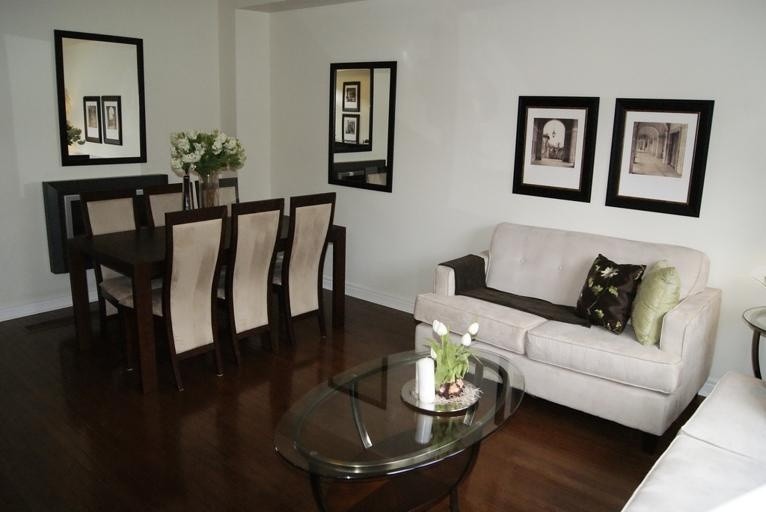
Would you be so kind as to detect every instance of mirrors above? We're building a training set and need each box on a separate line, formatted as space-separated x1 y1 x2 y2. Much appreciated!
327 59 399 195
332 67 373 154
53 27 147 165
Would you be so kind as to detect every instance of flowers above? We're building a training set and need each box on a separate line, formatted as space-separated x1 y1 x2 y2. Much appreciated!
428 319 483 385
170 128 241 176
65 119 85 148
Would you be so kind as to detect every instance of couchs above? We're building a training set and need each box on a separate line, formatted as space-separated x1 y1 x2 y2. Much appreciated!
611 372 766 512
406 221 725 437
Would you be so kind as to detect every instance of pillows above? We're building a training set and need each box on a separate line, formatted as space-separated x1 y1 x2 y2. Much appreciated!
628 264 685 348
572 246 643 337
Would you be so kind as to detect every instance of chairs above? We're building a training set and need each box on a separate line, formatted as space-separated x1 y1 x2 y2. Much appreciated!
31 168 238 338
285 186 337 361
227 195 289 365
96 205 225 394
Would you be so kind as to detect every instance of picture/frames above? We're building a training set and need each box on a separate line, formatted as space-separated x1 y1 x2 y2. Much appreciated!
342 114 360 147
100 96 124 147
603 95 717 219
342 82 361 112
81 92 102 145
510 94 601 203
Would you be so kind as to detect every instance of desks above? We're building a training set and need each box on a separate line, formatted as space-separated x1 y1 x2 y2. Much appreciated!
739 302 766 382
53 218 354 387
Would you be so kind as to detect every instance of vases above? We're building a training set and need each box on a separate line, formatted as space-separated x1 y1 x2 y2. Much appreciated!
192 163 222 210
433 372 468 400
